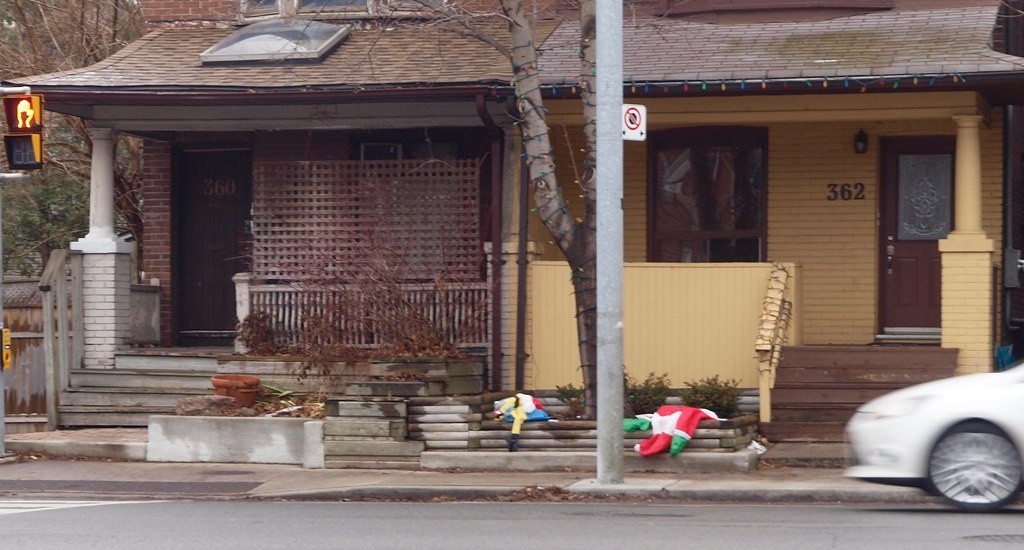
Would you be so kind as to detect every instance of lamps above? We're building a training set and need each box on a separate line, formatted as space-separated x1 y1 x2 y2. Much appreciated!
853 126 870 155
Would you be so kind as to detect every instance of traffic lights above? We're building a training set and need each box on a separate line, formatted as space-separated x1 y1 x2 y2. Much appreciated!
1 93 44 169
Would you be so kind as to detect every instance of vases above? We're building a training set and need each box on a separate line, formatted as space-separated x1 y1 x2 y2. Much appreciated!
211 375 261 409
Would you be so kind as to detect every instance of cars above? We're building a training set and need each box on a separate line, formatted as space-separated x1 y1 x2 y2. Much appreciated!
842 362 1024 513
